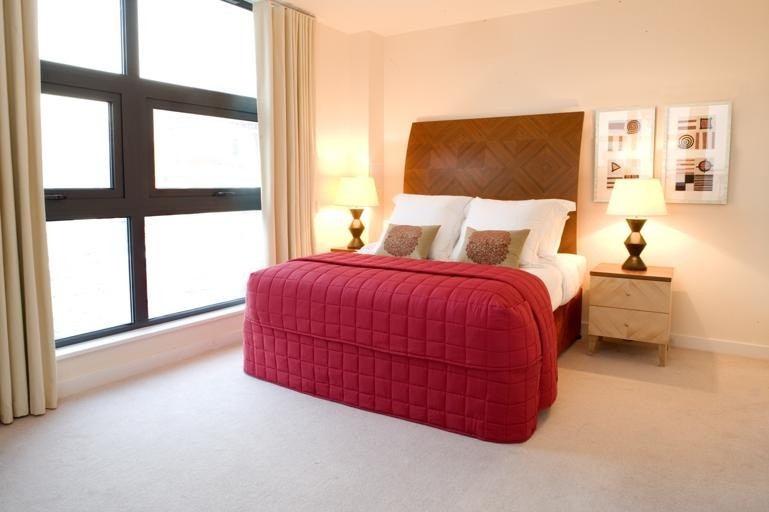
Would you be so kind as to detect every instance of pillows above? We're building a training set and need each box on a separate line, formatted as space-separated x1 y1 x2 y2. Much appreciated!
374 193 570 269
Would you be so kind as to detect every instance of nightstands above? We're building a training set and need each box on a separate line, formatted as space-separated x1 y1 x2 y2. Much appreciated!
585 263 678 367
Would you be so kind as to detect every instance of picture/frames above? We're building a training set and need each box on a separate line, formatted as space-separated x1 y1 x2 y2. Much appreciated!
590 99 732 205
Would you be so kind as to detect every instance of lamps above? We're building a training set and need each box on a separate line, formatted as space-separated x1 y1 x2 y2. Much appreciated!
337 172 380 250
603 179 669 273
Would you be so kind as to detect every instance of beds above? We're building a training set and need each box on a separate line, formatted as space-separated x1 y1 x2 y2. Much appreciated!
247 110 588 442
